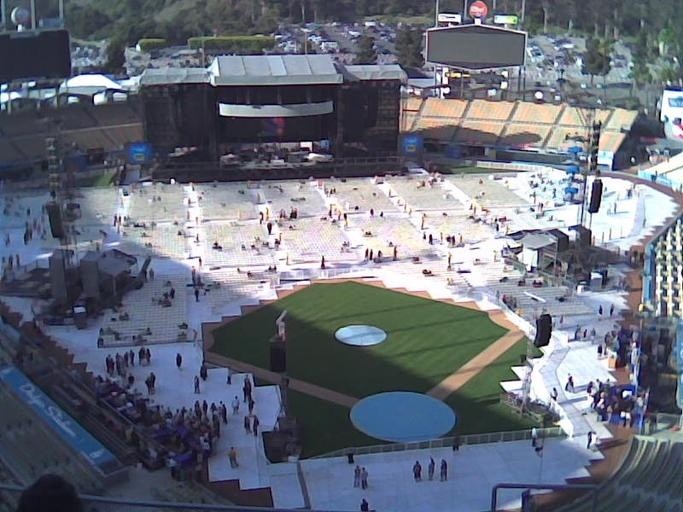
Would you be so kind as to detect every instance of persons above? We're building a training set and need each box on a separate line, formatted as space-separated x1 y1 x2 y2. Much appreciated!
352 464 361 488
259 169 637 294
18 474 82 512
428 455 435 480
413 460 422 482
495 294 649 454
1 183 260 476
360 498 369 511
360 466 368 488
452 434 461 452
439 458 447 481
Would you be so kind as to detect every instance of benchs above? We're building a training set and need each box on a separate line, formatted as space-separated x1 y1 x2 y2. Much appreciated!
0 336 201 479
644 219 683 318
